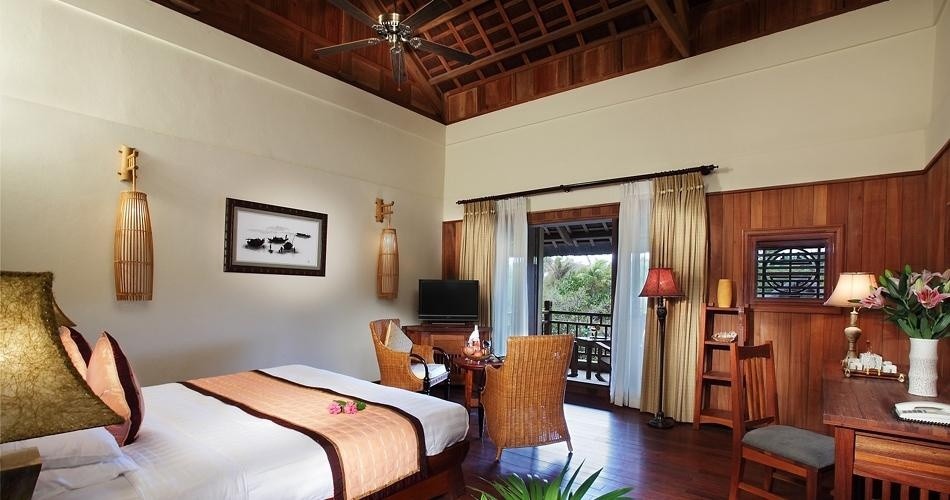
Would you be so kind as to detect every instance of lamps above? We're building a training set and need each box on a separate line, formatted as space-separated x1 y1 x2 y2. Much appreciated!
818 266 887 364
636 264 690 430
372 195 401 300
0 270 129 448
109 141 157 303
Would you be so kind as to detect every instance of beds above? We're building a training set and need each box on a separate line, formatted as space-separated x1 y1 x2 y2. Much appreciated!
0 362 480 497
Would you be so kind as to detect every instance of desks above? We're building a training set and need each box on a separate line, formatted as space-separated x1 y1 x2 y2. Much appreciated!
814 357 948 500
573 335 615 384
451 354 515 438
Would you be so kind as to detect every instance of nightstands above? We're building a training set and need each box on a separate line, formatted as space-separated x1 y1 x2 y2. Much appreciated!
1 443 43 500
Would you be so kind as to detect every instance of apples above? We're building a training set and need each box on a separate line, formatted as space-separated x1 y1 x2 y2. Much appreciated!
463 342 482 357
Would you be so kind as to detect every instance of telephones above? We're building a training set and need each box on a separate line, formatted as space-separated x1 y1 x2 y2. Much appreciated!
895 400 950 427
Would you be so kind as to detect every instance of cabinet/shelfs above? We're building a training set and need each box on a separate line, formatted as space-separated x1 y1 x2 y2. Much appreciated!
689 300 760 435
400 321 493 393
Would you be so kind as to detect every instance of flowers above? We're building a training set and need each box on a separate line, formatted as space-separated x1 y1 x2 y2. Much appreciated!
854 258 949 343
323 394 370 416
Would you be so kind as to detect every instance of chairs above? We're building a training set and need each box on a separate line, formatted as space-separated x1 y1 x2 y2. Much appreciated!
591 339 614 384
719 336 837 499
476 328 577 462
368 317 453 403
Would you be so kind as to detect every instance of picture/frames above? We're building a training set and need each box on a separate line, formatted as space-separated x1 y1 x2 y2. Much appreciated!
223 193 328 279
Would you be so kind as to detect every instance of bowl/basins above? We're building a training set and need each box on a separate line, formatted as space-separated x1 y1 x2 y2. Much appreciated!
463 346 491 362
711 332 737 342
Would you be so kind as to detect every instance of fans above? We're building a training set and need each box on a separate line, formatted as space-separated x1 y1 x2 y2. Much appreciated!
303 1 483 97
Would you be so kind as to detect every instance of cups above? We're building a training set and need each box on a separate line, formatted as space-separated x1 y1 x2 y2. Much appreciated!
717 279 733 308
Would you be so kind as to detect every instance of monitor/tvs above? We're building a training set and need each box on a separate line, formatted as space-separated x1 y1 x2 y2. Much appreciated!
418 279 480 323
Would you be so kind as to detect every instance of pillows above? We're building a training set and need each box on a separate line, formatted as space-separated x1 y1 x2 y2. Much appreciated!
53 315 92 383
1 422 130 476
83 326 145 446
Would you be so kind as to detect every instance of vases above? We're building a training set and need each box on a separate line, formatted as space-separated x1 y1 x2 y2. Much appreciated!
715 276 735 310
904 331 942 400
